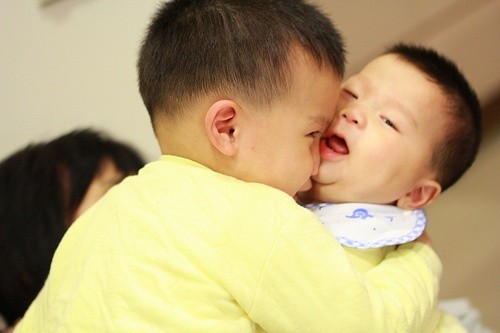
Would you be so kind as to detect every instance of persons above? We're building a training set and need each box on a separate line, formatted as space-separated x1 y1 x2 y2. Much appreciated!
13 0 442 333
0 127 145 333
295 42 484 333
